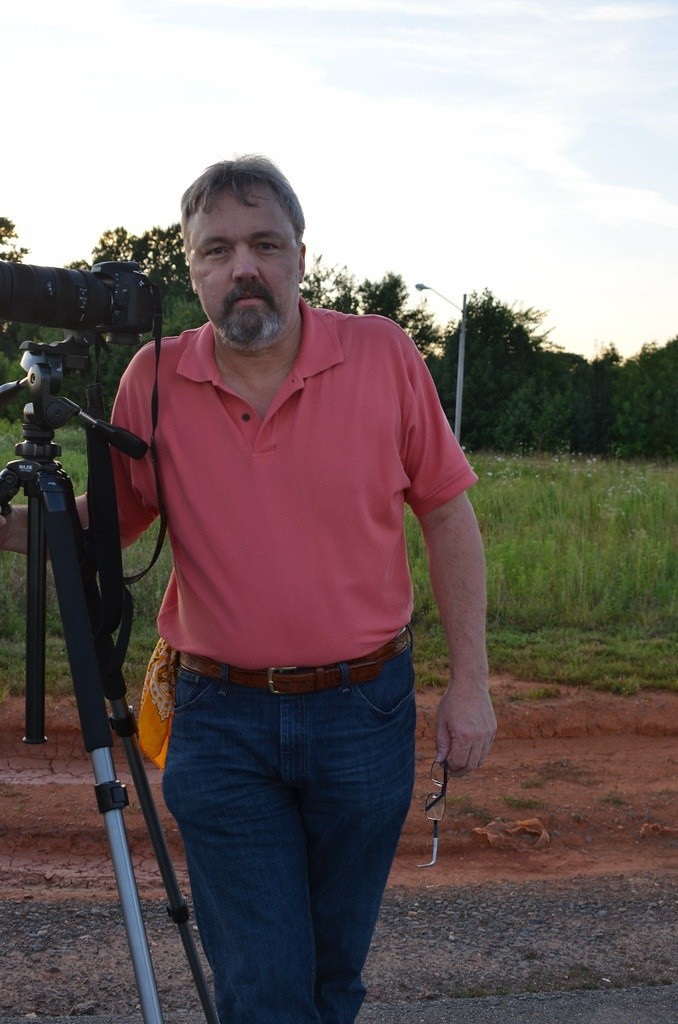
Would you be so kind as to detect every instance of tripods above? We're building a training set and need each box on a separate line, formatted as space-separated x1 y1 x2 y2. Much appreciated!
0 370 221 1024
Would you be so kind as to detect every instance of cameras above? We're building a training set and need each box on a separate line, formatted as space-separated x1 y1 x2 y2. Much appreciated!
0 257 156 345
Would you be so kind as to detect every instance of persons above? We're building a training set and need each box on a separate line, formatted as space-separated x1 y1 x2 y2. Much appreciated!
0 154 498 1024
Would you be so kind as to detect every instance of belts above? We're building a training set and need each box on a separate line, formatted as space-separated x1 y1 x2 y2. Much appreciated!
178 627 410 696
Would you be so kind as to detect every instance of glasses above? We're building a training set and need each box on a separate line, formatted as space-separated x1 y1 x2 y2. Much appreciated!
417 761 451 868
